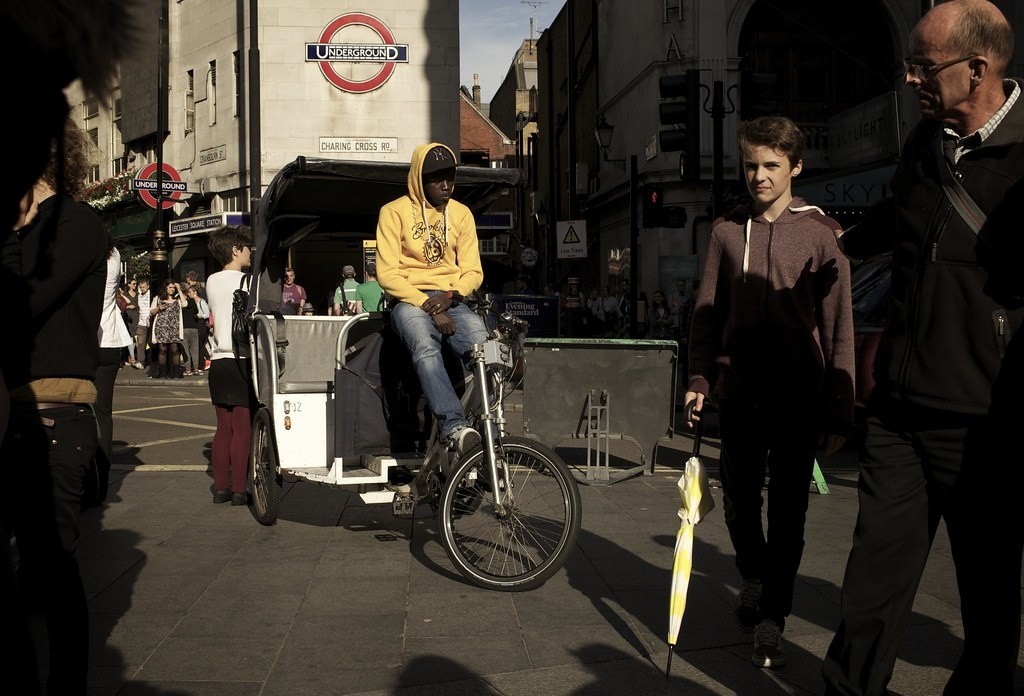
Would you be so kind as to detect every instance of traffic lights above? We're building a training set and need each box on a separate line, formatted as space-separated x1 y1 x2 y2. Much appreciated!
644 185 664 227
656 68 701 182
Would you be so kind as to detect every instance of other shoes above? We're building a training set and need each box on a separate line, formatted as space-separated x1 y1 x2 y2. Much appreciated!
132 361 144 370
183 369 192 376
203 358 211 371
231 493 252 506
193 370 203 376
213 493 232 504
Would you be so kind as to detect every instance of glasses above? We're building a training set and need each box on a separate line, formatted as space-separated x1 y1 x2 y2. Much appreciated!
904 53 986 83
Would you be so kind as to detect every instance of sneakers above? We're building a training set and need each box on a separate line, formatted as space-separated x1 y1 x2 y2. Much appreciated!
735 567 763 632
475 466 513 490
751 620 786 668
451 426 478 458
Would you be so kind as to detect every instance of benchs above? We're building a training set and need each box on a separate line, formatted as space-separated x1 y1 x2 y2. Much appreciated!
258 314 386 394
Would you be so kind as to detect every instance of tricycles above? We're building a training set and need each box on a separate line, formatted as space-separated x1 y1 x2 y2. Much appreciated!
254 155 581 595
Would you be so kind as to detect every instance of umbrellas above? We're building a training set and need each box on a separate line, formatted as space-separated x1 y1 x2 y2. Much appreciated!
666 399 715 677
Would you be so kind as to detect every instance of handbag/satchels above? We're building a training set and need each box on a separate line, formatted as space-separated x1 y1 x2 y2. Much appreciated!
232 278 256 344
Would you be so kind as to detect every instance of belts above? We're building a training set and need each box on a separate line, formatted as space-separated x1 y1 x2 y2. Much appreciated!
12 401 72 412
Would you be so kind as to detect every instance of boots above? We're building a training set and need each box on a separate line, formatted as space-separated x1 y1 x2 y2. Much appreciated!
156 364 167 381
173 364 182 381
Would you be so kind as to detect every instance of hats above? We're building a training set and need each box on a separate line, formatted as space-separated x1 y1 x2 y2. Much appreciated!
422 148 463 175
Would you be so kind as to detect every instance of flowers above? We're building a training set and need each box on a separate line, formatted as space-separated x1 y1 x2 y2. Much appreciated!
127 251 153 280
73 165 139 210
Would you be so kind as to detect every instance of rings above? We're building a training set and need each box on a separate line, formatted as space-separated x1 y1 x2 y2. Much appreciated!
437 304 441 308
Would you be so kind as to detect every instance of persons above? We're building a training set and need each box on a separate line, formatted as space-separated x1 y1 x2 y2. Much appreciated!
822 1 1023 696
205 226 261 506
283 267 307 315
0 0 135 696
375 142 514 493
327 264 385 316
683 115 856 668
516 276 700 390
116 266 209 379
